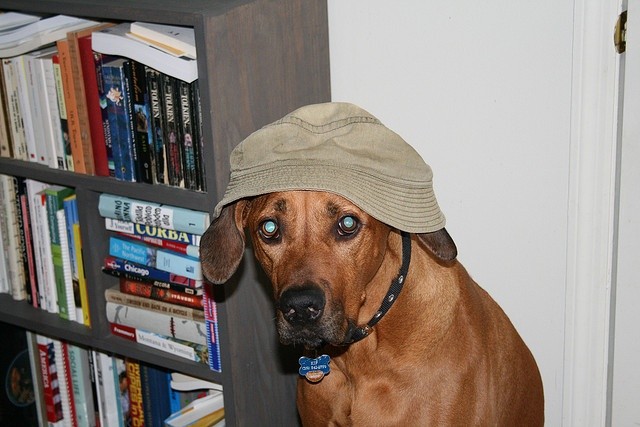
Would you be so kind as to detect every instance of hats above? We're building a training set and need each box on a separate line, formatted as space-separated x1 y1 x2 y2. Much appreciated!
212 102 447 233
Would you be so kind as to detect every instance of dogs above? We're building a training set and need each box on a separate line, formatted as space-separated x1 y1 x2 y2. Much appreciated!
198 102 544 427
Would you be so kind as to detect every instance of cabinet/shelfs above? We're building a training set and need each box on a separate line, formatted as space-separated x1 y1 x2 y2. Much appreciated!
0 2 329 427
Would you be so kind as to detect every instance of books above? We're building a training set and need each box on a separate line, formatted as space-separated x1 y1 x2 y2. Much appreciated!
0 320 227 427
97 192 223 372
0 10 207 194
2 174 89 328
